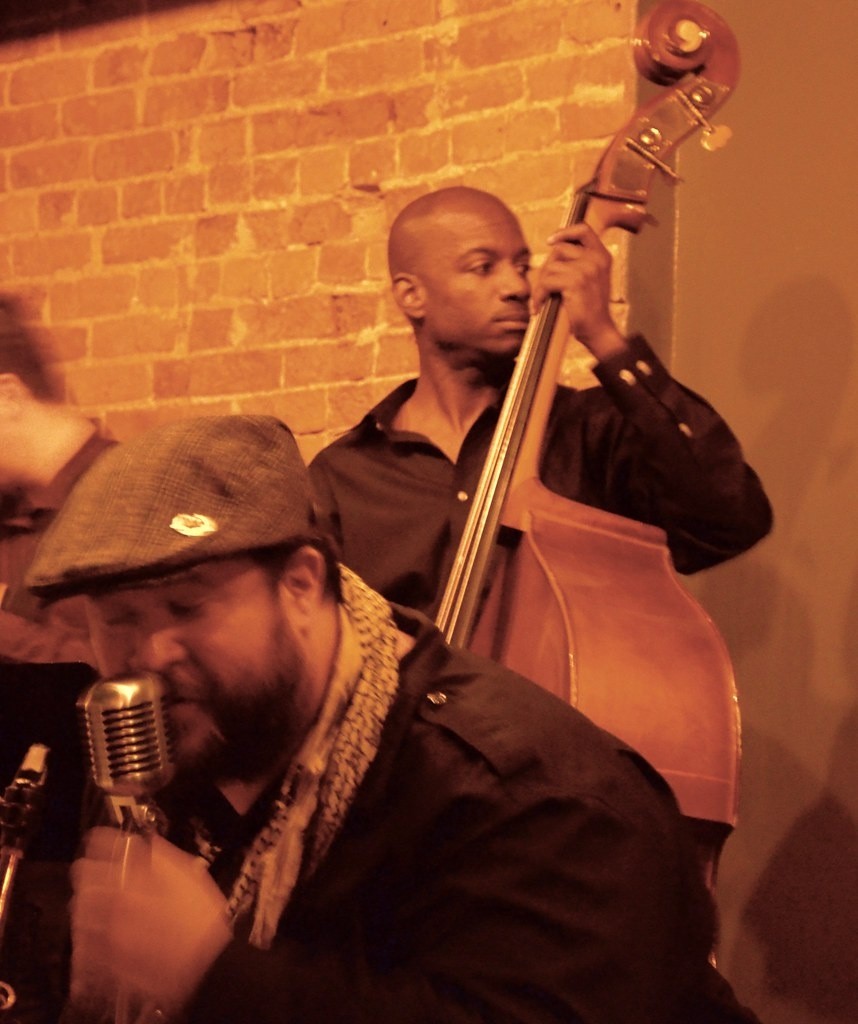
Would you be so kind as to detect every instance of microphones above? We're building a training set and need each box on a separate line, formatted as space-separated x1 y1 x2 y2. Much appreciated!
78 671 175 837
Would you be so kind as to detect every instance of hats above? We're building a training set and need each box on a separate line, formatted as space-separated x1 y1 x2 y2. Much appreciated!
26 415 313 602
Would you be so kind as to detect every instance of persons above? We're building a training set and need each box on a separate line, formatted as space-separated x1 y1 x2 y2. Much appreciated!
0 290 124 676
305 184 776 626
0 412 763 1024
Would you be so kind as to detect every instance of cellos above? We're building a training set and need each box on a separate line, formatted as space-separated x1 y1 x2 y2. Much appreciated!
430 2 743 965
